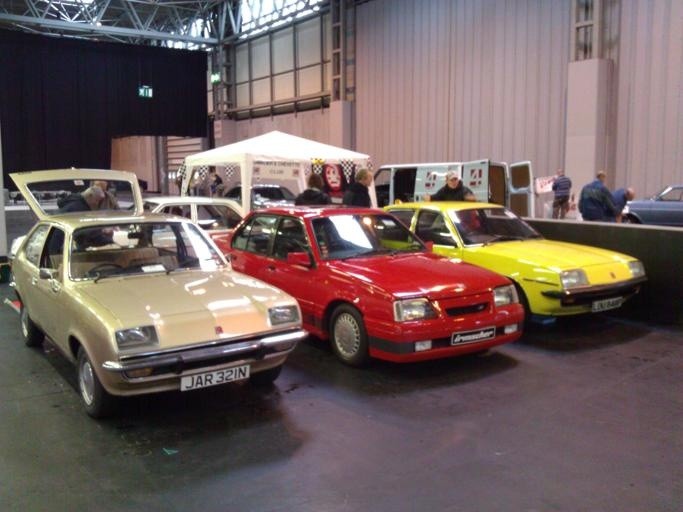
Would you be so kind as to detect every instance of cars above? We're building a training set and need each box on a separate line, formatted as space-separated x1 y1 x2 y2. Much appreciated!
377 200 652 328
620 183 683 228
6 169 310 421
209 203 525 369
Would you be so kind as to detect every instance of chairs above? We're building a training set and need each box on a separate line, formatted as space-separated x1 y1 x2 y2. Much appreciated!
43 248 178 276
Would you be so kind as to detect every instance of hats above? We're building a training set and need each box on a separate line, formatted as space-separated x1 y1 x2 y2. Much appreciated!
446 172 458 181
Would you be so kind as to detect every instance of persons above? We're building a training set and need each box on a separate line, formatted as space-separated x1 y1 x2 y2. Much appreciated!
421 170 477 203
46 185 128 255
291 174 332 228
208 167 224 194
87 180 120 212
610 185 635 224
342 168 374 209
551 168 572 219
578 169 628 222
